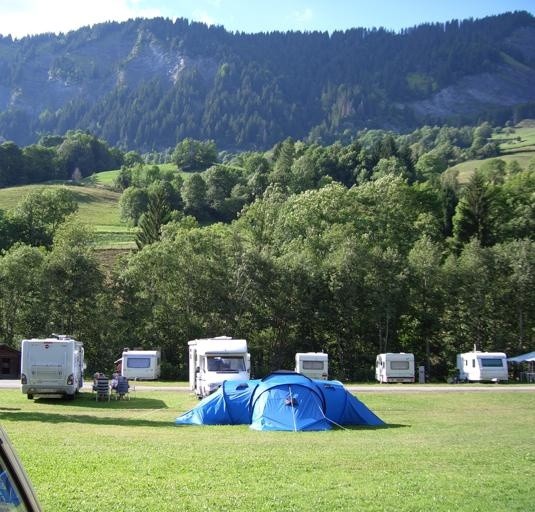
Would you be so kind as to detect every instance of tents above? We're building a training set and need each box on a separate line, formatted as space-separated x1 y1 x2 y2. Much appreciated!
176 369 386 432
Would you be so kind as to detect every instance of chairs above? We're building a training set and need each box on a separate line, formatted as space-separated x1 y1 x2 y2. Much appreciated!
92 376 139 401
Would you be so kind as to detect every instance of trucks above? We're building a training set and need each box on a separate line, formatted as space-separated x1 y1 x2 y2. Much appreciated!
21 332 85 400
189 334 252 398
295 351 329 383
377 351 416 386
457 351 509 383
121 350 161 380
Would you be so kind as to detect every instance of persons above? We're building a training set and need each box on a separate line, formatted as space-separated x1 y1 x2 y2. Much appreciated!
92 363 130 401
